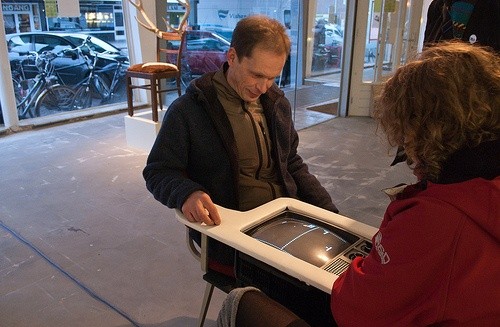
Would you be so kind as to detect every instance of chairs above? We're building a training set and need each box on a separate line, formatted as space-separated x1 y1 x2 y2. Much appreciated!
174 198 379 327
124 0 190 122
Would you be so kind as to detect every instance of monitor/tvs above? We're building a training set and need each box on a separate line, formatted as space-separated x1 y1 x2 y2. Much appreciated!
245 212 360 268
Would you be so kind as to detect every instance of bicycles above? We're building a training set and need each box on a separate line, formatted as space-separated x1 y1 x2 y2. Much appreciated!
0 35 130 124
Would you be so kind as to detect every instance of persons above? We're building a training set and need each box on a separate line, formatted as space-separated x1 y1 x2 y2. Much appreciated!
142 16 339 327
216 38 500 327
391 0 500 166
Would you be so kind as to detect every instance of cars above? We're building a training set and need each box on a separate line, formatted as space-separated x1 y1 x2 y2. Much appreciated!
166 30 231 81
4 31 130 100
311 18 343 75
